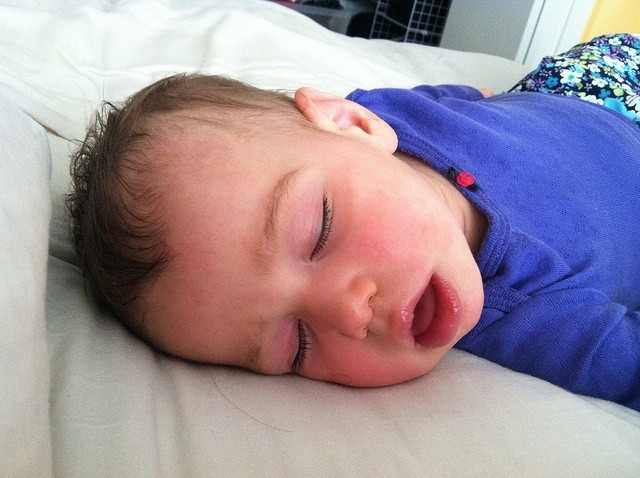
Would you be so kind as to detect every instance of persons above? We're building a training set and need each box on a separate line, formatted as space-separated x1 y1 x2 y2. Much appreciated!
71 33 640 414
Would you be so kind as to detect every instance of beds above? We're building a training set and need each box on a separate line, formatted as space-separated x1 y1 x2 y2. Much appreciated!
0 1 638 473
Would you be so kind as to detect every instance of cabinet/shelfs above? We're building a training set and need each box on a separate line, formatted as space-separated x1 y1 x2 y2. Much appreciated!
440 0 597 70
367 0 451 46
